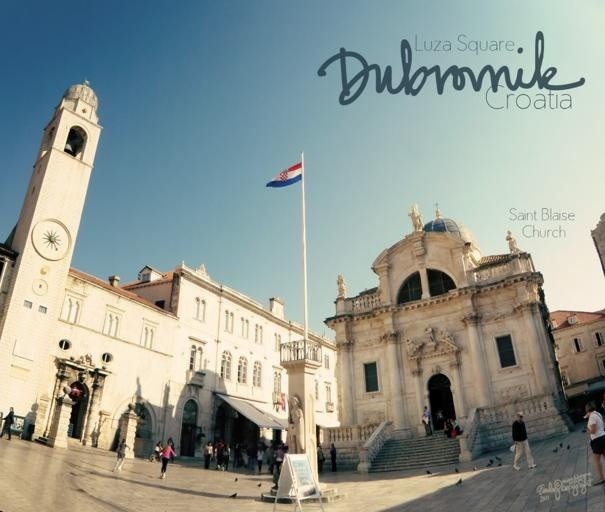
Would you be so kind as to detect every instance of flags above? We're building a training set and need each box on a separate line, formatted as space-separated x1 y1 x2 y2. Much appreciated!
266 161 302 188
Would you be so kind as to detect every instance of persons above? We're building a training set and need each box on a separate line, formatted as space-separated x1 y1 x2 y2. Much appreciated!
291 396 305 454
0 407 14 440
317 443 325 472
583 403 605 485
409 209 422 230
422 405 463 438
512 412 536 471
337 274 345 297
506 230 519 254
112 439 130 472
155 437 177 479
114 428 121 450
204 440 287 474
330 443 337 472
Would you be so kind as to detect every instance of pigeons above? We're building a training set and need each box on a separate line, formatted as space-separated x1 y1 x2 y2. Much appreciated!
426 470 432 474
473 464 477 471
235 477 238 482
486 456 502 467
582 429 587 433
455 478 462 487
454 467 460 473
256 483 261 488
552 442 570 452
229 493 237 499
